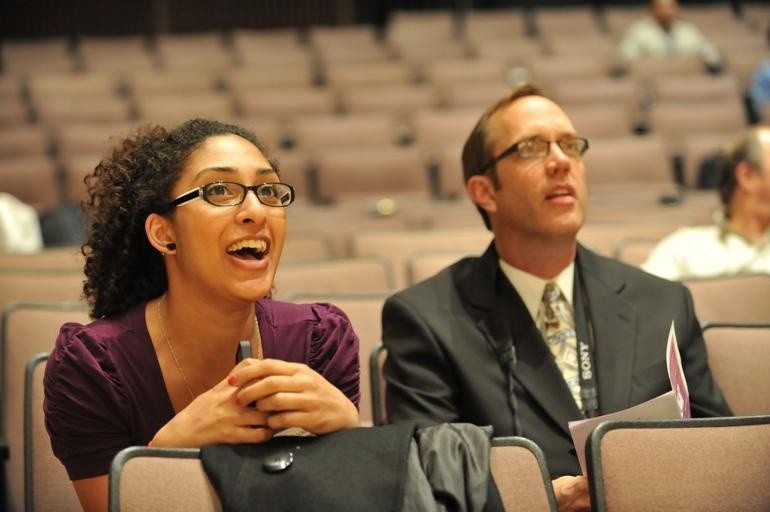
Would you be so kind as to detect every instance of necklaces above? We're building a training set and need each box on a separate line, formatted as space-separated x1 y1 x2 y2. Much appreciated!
157 293 260 405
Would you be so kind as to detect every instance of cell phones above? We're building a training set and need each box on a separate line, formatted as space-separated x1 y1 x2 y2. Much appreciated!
234 341 251 365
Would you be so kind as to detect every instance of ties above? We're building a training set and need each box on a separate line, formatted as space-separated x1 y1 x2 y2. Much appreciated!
543 283 583 414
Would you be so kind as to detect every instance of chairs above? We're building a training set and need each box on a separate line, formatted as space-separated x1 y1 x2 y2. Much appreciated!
109 433 555 511
21 355 80 508
1 0 769 421
588 417 770 512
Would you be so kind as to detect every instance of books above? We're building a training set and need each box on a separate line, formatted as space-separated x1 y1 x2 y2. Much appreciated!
566 315 695 486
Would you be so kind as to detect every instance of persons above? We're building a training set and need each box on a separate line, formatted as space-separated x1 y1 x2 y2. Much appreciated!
635 123 770 283
382 84 733 512
744 25 769 122
617 1 721 76
41 120 361 510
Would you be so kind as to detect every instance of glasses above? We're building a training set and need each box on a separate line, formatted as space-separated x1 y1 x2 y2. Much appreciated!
482 134 588 173
164 181 294 208
